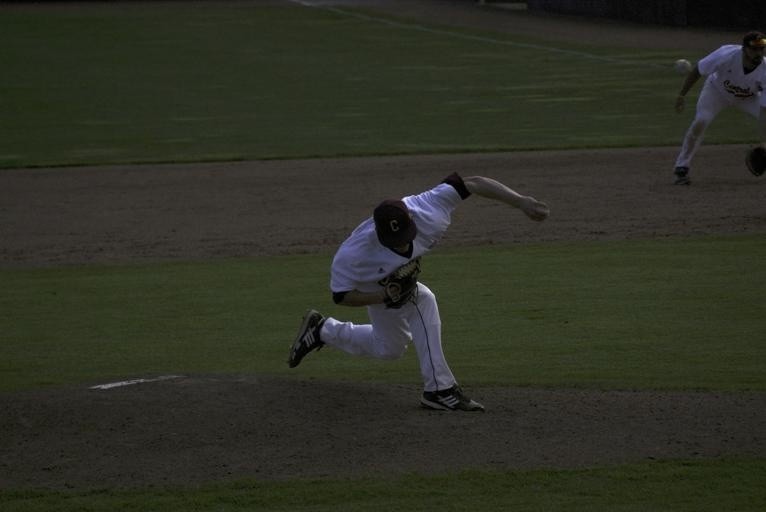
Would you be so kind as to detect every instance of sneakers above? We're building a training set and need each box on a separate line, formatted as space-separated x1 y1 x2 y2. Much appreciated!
419 387 486 413
285 308 325 368
672 171 692 185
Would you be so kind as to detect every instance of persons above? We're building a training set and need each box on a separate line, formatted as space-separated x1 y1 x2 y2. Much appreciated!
670 30 765 187
286 168 550 415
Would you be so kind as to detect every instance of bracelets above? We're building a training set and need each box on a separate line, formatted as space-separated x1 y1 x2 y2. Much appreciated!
678 94 684 98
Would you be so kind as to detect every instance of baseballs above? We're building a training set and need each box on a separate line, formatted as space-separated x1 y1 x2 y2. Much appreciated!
534 199 550 217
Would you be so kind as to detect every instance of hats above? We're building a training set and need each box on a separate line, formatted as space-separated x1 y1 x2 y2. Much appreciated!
742 29 766 50
372 200 419 248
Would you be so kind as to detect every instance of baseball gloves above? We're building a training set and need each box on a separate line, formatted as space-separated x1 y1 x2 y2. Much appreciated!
383 259 422 307
745 146 766 176
